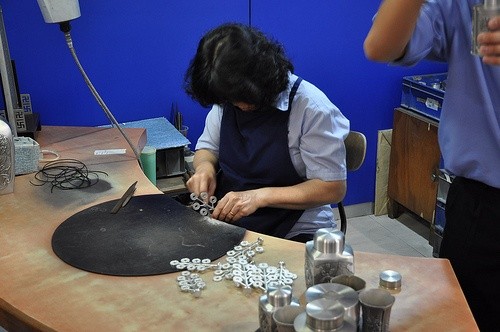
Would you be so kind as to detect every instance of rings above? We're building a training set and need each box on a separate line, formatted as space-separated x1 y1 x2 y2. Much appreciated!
230 212 235 216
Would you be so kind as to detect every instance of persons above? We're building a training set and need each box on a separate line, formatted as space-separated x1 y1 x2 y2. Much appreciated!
185 23 349 244
363 0 500 332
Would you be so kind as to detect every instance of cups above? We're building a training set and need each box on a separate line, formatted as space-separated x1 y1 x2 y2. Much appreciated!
470 1 500 58
13 135 39 177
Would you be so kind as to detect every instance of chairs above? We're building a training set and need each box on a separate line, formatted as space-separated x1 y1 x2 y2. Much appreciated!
333 131 366 234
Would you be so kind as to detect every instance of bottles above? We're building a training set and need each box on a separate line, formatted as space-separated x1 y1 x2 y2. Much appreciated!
0 119 15 195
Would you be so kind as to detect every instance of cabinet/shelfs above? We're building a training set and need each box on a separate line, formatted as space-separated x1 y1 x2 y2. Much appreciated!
95 117 191 198
387 108 440 245
434 175 452 240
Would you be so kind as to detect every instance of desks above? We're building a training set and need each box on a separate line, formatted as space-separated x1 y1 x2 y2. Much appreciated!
0 125 480 332
